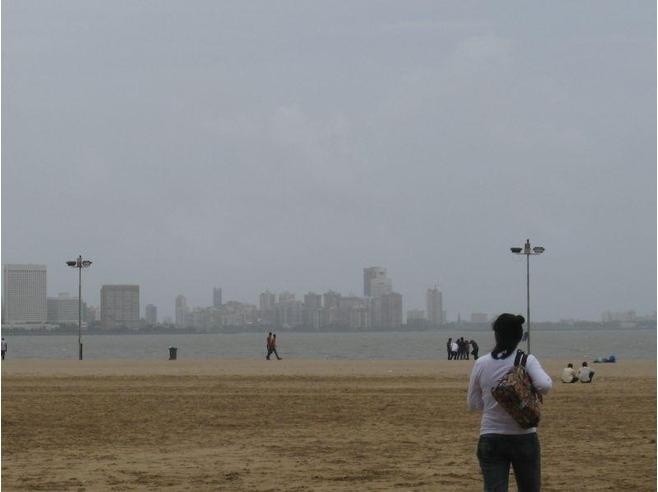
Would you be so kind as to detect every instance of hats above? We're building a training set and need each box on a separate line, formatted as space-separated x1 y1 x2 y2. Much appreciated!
494 314 524 329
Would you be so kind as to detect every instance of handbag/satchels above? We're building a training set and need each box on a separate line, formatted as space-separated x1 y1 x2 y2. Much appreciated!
491 349 542 429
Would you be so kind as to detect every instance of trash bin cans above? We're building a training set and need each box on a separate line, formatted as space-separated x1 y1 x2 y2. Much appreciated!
168 346 177 360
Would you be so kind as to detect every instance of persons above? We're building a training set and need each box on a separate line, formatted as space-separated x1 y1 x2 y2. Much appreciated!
577 361 595 383
267 331 272 350
447 336 479 360
467 313 553 492
266 334 282 360
1 338 8 359
561 363 579 383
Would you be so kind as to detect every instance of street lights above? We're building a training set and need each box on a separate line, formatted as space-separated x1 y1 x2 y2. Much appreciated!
65 255 93 360
511 239 545 354
